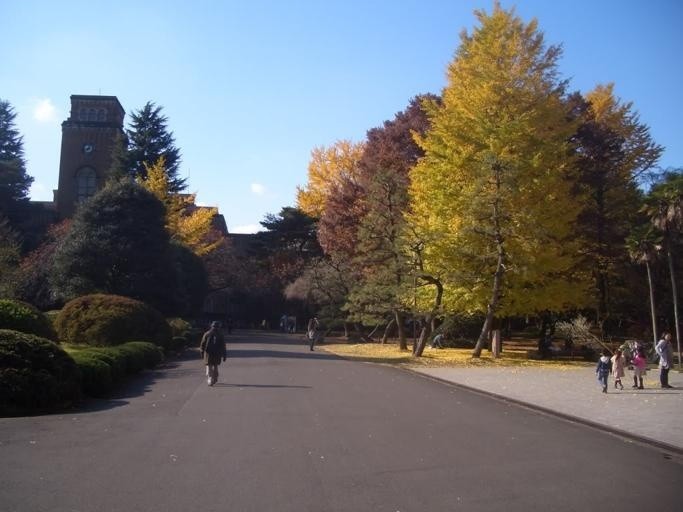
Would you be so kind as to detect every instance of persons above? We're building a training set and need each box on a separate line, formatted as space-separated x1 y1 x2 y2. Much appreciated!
431 334 446 348
655 329 674 389
630 346 646 389
308 317 318 351
611 348 624 390
199 320 225 385
596 348 610 393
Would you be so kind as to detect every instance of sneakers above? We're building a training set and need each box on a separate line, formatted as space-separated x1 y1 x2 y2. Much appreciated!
662 384 672 388
603 387 607 394
208 377 217 386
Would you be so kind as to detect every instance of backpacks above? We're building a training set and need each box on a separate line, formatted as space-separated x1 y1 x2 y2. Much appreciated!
205 334 220 353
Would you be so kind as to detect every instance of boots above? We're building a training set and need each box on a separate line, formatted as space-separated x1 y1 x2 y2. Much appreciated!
632 376 643 389
614 380 624 390
